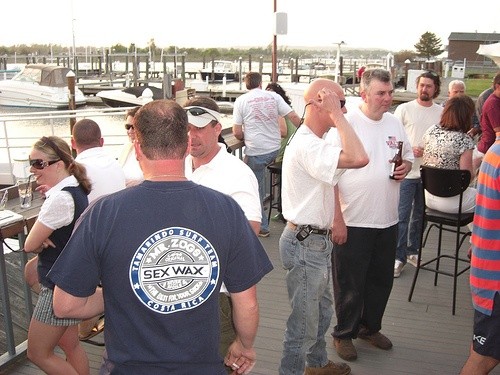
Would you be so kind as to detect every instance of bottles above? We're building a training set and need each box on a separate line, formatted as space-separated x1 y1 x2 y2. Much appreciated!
390 141 404 181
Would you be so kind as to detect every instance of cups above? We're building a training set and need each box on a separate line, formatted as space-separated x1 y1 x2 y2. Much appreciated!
17 180 32 209
0 189 8 211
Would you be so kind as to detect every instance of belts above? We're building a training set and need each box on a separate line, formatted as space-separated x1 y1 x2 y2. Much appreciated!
311 228 332 236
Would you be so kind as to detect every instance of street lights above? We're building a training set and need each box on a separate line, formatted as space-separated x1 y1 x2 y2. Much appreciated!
73 18 76 67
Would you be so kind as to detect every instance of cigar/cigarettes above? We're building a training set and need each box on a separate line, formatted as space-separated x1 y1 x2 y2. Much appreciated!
228 360 240 369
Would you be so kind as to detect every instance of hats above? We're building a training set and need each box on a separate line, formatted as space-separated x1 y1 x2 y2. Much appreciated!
182 105 224 128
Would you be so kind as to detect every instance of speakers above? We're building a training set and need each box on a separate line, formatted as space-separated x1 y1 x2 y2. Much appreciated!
273 12 287 37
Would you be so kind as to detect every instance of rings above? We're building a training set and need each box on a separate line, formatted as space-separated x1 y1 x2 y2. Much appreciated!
406 173 407 175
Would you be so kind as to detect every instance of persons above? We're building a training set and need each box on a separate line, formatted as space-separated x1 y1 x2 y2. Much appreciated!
24 135 92 375
120 106 145 188
441 80 466 108
233 72 302 237
266 83 298 221
474 74 500 158
461 130 500 375
183 97 262 235
422 95 476 243
326 68 415 360
279 78 370 375
46 100 275 375
394 72 444 277
24 119 127 342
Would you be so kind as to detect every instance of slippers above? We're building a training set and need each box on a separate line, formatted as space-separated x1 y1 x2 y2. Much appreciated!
79 315 106 342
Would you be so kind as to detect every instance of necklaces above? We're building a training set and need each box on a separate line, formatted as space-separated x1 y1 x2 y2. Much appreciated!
148 175 185 178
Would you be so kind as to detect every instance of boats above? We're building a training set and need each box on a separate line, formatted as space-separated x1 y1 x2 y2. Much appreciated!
0 64 88 109
95 84 164 108
198 60 240 83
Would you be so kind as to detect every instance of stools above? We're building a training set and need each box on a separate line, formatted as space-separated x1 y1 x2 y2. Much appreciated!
408 165 475 316
267 161 286 226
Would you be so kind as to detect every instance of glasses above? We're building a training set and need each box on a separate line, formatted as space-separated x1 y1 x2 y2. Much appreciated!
28 155 62 169
125 123 136 131
340 99 345 108
183 107 218 122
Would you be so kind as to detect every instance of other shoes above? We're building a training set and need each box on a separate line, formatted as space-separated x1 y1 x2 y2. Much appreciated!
271 212 282 221
333 336 357 360
393 259 407 277
304 359 351 375
258 230 270 238
363 331 393 350
406 252 419 267
